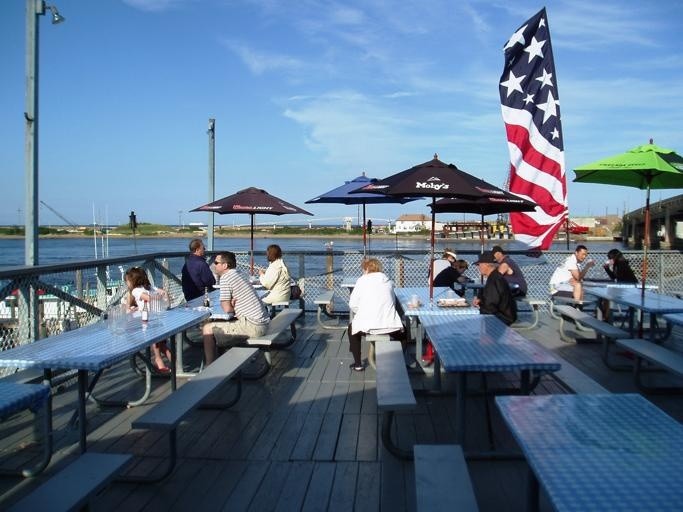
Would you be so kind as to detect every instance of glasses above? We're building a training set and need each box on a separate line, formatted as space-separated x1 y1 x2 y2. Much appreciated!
215 261 223 264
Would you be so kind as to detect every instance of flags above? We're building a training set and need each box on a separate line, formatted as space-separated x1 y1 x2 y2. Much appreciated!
498 9 570 253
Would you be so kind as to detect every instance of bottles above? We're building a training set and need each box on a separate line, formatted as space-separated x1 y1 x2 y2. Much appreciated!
140 300 150 322
142 322 148 343
203 287 210 308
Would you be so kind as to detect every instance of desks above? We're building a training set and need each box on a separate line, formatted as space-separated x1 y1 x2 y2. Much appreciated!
392 286 482 368
566 276 682 335
166 288 272 359
342 277 355 330
417 313 564 462
0 309 213 441
494 393 682 512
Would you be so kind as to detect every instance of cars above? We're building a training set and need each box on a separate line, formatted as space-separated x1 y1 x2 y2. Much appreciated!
557 221 589 234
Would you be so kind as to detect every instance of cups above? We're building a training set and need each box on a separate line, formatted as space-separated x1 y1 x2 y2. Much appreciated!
602 261 610 269
148 291 165 320
447 291 455 299
98 303 126 335
588 259 595 270
209 301 215 307
411 294 420 308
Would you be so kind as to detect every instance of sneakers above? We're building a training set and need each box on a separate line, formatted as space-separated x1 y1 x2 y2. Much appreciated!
350 362 363 370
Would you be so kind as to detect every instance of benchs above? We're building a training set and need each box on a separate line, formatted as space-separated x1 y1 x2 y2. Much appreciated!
366 331 390 373
540 351 613 395
617 337 682 379
271 293 306 320
411 444 479 512
517 298 547 331
0 380 55 510
314 288 337 330
0 449 134 510
244 308 305 377
372 340 419 459
553 303 629 371
130 344 261 485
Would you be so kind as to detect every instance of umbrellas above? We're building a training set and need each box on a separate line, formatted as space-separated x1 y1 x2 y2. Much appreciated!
428 180 540 256
303 170 426 255
349 152 524 253
187 186 314 276
571 137 683 294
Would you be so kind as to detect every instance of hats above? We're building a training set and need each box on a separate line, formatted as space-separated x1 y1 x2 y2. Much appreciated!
472 253 498 265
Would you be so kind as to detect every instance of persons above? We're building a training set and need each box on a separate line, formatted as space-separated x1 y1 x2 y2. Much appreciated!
181 239 216 301
258 245 290 313
426 248 456 285
201 252 270 369
345 258 408 371
471 251 518 326
432 259 469 299
547 245 595 308
125 266 171 370
491 245 527 297
598 248 638 324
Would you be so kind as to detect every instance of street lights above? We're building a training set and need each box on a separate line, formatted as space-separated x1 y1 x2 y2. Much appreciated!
16 0 65 346
205 118 215 251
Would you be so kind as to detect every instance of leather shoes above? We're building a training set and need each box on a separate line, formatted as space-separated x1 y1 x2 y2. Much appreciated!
154 360 169 372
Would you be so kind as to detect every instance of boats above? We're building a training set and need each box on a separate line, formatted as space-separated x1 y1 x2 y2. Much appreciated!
424 218 578 245
0 200 138 322
